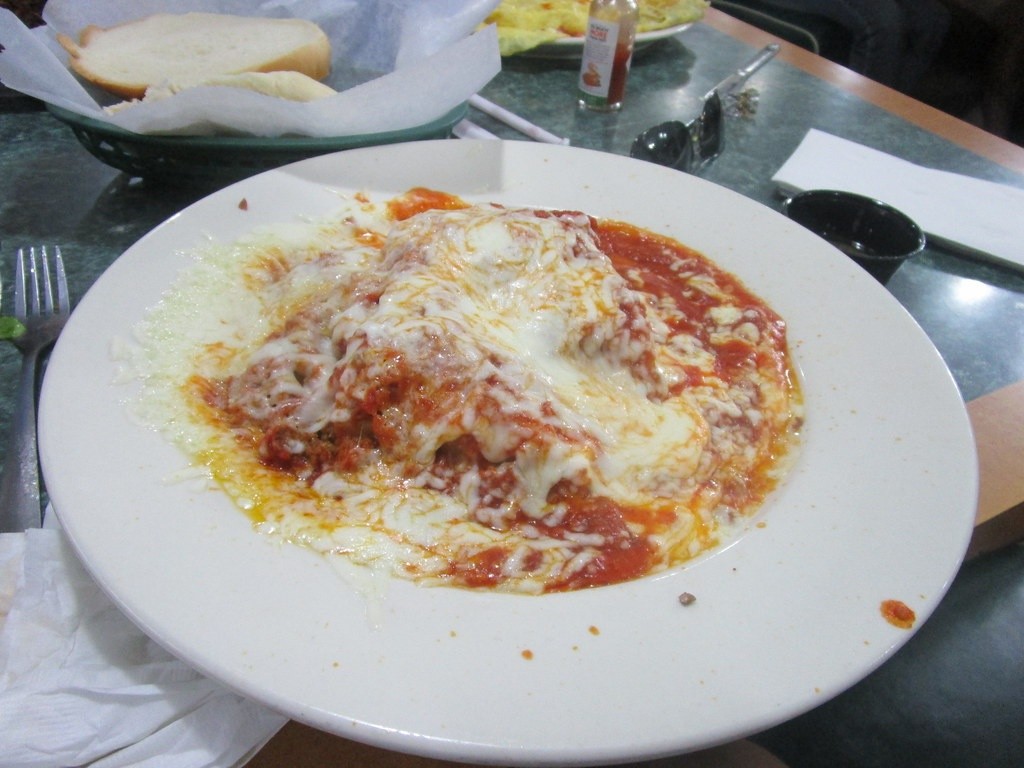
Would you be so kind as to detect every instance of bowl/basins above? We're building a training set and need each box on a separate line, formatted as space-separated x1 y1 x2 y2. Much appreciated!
783 190 926 284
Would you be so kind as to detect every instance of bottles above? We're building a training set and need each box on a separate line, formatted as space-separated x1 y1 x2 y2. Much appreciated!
577 0 638 111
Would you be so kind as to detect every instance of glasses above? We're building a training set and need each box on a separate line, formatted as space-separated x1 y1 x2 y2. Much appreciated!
630 88 725 175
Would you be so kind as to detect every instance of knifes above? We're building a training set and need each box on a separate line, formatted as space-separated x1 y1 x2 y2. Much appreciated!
704 44 780 101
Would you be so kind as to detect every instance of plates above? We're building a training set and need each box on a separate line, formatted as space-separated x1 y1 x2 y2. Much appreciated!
516 0 711 60
38 139 980 762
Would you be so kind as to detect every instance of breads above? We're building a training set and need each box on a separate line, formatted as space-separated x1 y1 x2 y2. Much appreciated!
57 12 332 96
102 69 338 115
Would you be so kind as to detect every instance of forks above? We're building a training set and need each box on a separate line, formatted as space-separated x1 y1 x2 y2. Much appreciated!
13 245 70 532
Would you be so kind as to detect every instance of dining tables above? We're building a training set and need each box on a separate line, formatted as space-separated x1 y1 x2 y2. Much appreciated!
0 0 1024 768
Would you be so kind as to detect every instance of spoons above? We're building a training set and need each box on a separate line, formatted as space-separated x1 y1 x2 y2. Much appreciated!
632 122 689 169
692 94 726 176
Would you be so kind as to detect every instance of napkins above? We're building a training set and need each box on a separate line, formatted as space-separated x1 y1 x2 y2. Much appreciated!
772 128 1024 275
0 502 291 768
